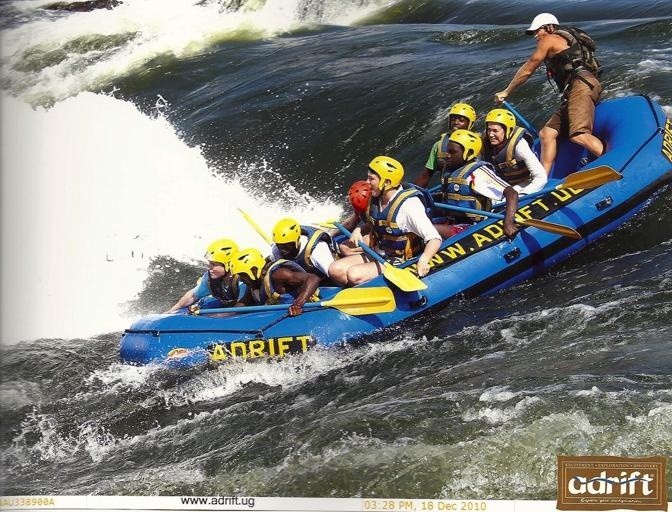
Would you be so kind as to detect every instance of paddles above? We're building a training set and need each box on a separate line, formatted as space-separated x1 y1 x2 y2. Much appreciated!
191 286 395 316
326 217 428 292
237 207 275 249
434 202 582 241
491 165 623 210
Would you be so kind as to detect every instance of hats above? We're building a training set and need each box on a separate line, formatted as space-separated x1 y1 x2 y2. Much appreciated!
524 13 560 35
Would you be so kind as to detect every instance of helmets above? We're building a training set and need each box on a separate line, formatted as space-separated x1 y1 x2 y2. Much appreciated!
484 108 516 140
272 218 301 258
203 238 240 273
348 181 371 214
368 156 404 191
449 129 482 162
230 247 267 280
449 103 477 130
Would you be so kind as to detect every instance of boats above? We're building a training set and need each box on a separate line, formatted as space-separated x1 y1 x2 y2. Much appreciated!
115 90 672 367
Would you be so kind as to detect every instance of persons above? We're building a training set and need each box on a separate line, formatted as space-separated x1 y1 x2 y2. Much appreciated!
493 12 606 176
161 102 548 316
